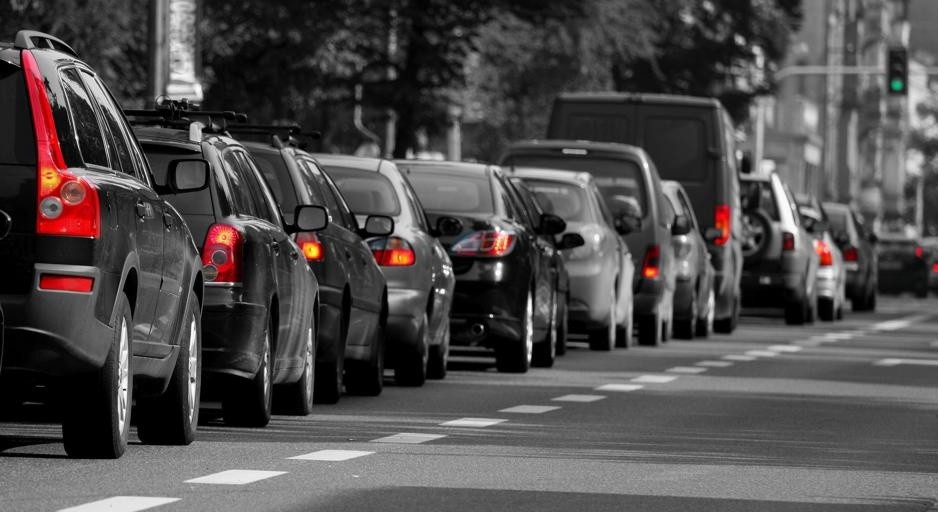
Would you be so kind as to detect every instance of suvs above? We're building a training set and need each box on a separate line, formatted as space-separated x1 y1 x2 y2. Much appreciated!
1 29 211 460
736 164 818 325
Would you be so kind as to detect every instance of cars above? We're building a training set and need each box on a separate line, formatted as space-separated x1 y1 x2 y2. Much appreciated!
794 191 937 322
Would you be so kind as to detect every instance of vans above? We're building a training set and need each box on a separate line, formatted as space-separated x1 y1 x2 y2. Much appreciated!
547 91 746 332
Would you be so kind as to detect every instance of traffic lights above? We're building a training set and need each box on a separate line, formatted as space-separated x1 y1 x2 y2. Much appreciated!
888 49 908 95
